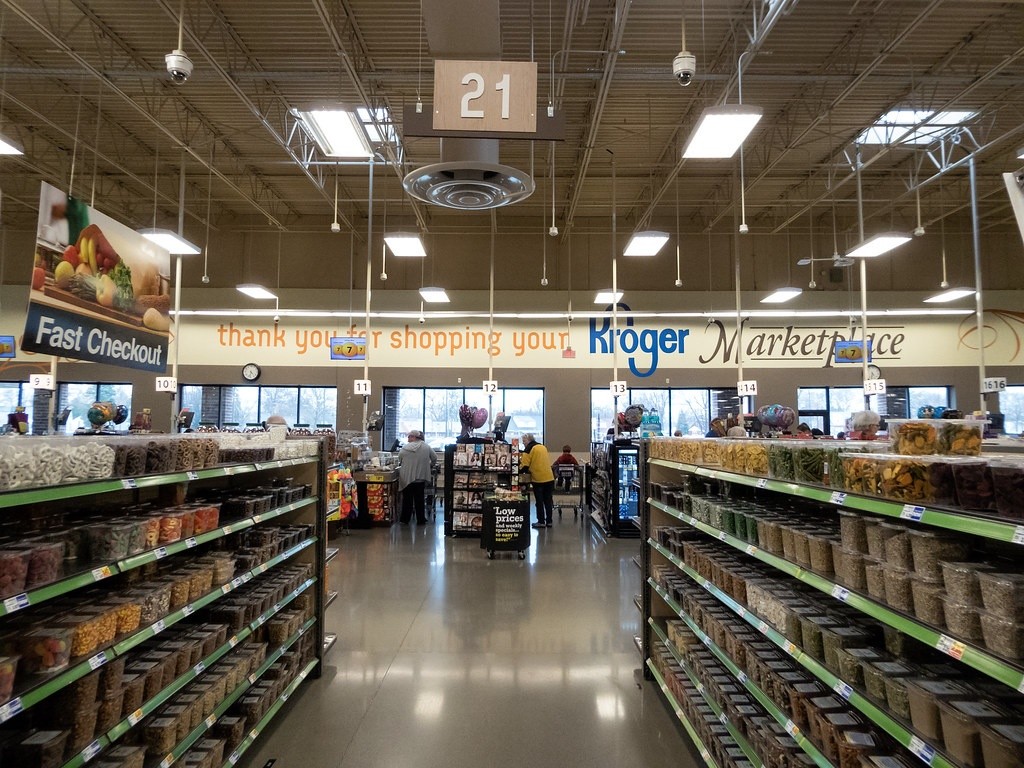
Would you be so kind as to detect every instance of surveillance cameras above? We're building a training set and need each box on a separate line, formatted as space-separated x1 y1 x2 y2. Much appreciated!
417 318 425 324
672 56 696 85
164 55 192 85
274 316 280 323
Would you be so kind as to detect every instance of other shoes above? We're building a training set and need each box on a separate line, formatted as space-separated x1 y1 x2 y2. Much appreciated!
546 524 552 527
532 522 546 527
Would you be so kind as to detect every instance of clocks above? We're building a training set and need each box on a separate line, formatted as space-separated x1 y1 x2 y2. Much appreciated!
242 362 260 382
862 365 881 380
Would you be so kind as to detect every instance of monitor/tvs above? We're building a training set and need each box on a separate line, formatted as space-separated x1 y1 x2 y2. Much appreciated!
391 439 400 452
0 336 15 358
494 416 511 433
366 414 386 432
57 409 71 425
986 414 1005 429
743 417 763 431
177 411 195 429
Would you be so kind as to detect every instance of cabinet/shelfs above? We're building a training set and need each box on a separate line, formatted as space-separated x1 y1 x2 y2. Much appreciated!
588 440 639 540
326 430 374 521
631 434 1024 768
443 442 512 541
0 432 339 768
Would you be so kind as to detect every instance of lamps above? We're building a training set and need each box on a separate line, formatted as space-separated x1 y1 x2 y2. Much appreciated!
0 51 975 304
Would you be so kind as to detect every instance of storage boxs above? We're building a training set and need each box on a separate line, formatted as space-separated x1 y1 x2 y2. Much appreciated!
886 417 993 455
0 432 318 768
645 481 1024 768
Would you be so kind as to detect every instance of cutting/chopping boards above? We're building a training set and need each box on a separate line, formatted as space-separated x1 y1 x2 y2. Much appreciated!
44 285 144 326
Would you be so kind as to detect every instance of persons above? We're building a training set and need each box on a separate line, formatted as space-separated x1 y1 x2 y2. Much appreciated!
487 458 493 464
797 410 881 440
469 452 482 466
456 492 465 506
398 430 438 525
518 433 555 528
705 418 726 437
551 445 580 492
472 492 482 504
498 454 510 470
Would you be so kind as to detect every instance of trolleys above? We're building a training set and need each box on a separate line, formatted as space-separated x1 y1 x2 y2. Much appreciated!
550 463 584 517
411 463 441 522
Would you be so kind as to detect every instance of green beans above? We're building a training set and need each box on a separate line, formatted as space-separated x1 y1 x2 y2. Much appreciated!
766 445 869 492
942 424 963 440
